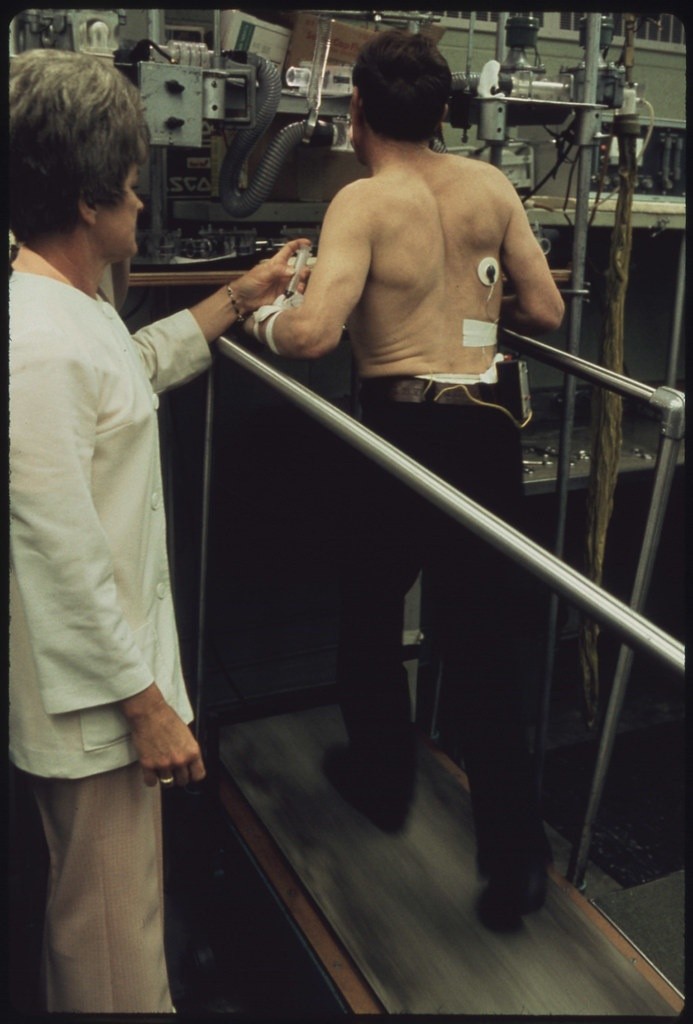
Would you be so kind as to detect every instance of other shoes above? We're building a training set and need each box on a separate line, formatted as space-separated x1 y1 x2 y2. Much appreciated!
324 741 409 835
476 857 546 916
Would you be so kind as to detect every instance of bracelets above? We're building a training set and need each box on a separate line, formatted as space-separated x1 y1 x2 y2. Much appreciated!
226 280 245 323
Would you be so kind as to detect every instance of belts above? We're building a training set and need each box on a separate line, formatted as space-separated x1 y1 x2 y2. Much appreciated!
363 379 498 407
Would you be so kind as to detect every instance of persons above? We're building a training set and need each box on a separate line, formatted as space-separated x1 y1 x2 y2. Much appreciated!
242 31 566 924
0 43 316 1015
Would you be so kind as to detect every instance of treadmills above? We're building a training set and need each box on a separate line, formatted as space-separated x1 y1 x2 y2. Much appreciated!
187 678 689 1016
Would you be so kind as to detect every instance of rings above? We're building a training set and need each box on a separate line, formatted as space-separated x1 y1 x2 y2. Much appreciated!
160 776 174 784
